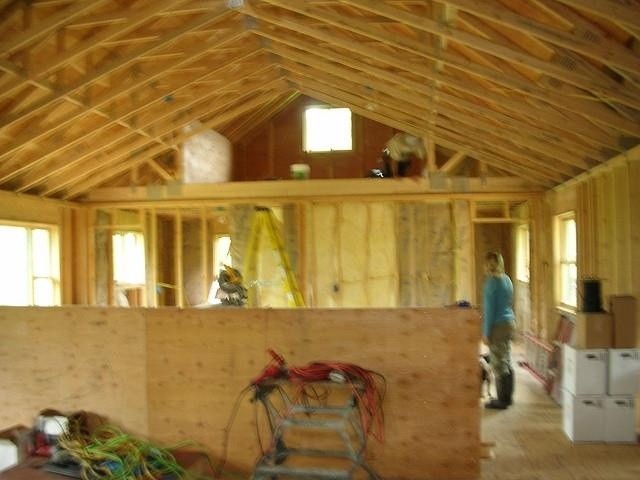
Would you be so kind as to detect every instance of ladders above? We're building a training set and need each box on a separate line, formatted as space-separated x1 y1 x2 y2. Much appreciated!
242 204 305 309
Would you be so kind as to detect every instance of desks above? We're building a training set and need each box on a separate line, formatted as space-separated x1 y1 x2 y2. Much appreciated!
0 444 208 480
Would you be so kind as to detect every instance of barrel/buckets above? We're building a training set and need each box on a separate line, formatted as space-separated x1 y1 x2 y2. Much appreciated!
289 163 311 181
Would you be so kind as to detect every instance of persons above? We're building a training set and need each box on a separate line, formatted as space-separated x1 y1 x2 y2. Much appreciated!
479 352 494 399
364 131 426 178
482 251 518 410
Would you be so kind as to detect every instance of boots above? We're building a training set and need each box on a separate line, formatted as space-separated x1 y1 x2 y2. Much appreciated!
492 374 513 409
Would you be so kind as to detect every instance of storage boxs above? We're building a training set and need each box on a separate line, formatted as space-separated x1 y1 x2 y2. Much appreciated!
561 295 640 443
